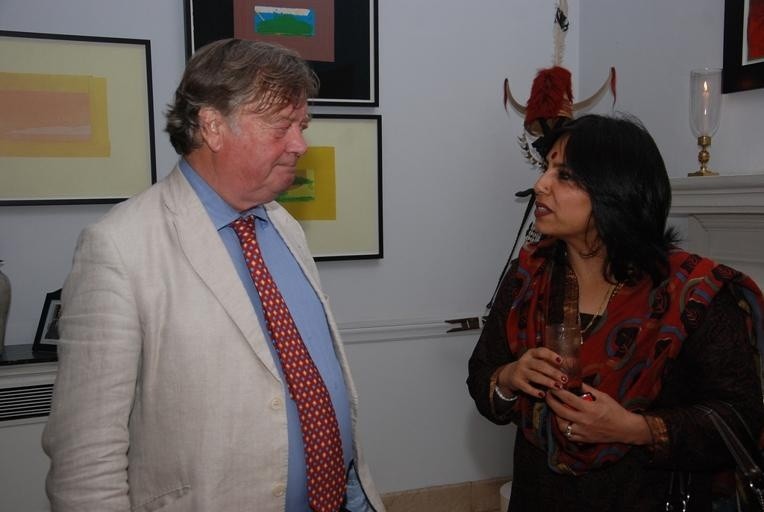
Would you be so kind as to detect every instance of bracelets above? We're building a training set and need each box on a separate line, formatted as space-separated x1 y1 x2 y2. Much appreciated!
495 387 519 402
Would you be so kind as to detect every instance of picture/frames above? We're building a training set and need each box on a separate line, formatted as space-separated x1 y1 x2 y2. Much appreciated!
0 30 157 206
271 114 383 261
184 0 379 107
32 288 61 352
721 0 764 94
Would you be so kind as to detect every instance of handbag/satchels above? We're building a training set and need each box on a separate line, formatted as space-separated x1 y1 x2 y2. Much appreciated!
664 400 763 511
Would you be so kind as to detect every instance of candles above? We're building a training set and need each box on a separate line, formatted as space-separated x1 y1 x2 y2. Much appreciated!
700 81 709 133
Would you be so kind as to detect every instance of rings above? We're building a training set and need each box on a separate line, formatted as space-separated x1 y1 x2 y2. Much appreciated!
564 424 572 438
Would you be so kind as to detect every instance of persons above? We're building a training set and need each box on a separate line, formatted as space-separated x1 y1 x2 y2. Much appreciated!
464 112 762 512
44 40 388 512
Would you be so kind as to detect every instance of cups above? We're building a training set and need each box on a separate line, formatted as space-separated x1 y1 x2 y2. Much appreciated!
544 324 583 413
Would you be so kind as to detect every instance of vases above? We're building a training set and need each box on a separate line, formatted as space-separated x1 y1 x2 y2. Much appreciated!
0 260 11 354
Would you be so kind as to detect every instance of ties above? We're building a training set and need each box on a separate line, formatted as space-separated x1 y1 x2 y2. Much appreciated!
231 215 347 512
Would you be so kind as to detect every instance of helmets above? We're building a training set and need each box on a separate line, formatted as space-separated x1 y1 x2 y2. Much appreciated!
503 67 614 169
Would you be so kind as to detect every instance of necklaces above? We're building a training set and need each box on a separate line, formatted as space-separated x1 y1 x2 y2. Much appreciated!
568 258 618 346
